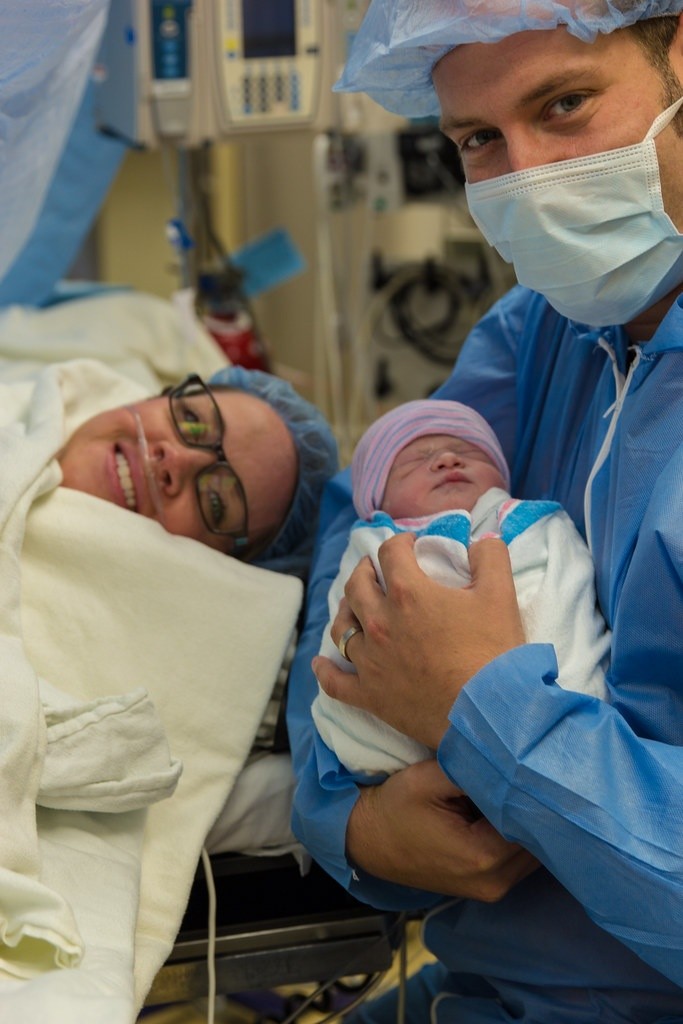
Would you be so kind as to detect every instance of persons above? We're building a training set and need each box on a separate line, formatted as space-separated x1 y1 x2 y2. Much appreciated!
288 0 683 1024
59 365 340 582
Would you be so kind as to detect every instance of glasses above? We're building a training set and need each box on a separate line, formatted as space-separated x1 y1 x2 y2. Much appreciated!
161 371 248 561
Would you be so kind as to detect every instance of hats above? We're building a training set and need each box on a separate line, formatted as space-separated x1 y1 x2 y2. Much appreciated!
351 399 510 521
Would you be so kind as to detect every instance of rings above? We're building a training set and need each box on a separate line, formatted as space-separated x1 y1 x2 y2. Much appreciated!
339 627 363 662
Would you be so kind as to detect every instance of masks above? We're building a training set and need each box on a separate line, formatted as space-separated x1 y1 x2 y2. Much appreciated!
464 97 683 327
